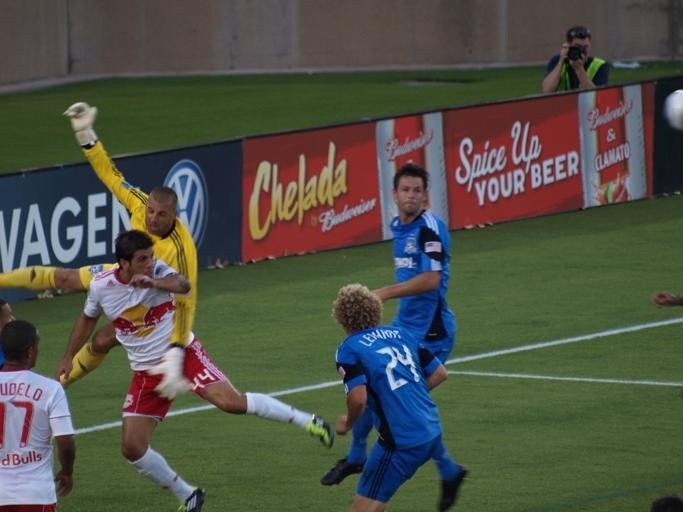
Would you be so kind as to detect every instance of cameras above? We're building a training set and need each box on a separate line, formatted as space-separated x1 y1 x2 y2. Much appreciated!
564 44 586 64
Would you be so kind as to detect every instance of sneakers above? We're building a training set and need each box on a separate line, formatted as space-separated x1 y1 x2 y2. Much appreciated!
438 465 469 511
176 487 206 512
320 456 363 487
305 412 334 449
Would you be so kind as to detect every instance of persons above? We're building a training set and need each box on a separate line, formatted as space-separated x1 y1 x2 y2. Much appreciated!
0 101 199 402
542 24 609 95
0 298 15 369
54 228 334 512
328 282 451 512
0 320 76 511
318 162 471 511
651 289 683 308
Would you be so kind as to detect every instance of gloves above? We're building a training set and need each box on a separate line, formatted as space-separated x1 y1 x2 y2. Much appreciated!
148 347 192 401
62 100 98 148
